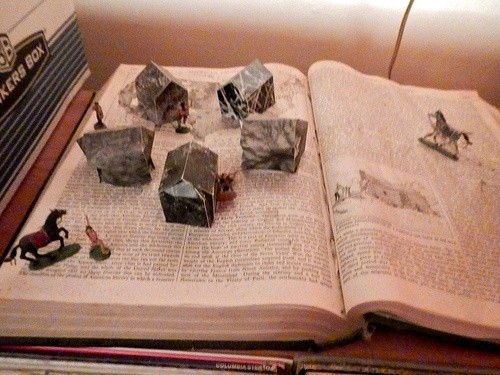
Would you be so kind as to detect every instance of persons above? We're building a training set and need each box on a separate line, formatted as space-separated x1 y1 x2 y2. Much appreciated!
86 224 112 255
220 171 236 193
93 100 106 127
175 100 191 130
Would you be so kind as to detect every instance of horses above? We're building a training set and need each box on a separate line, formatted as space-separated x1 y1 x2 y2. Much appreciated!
4 208 69 265
418 110 473 159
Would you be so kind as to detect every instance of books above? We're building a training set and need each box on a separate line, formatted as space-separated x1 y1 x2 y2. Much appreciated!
2 59 500 351
